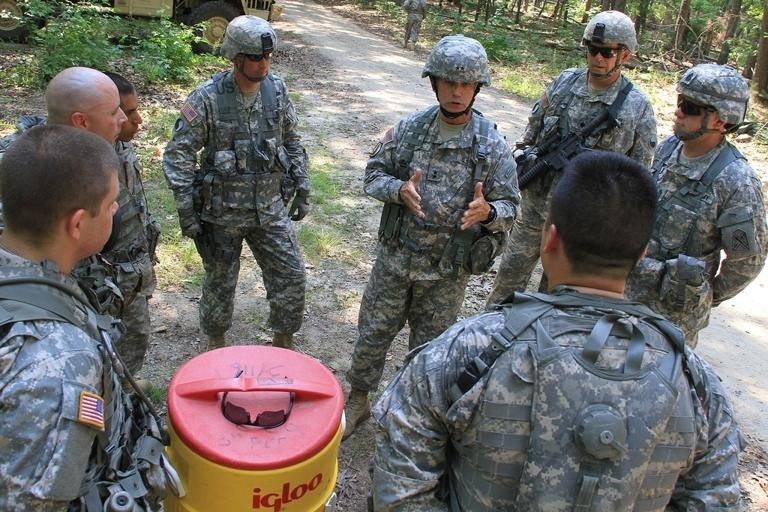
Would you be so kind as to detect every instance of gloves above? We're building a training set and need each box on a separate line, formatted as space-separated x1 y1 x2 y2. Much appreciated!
180 214 203 238
288 188 310 221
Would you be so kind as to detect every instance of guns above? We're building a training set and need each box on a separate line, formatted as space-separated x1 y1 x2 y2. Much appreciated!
514 106 622 191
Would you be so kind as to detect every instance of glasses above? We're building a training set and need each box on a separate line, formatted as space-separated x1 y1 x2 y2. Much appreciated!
241 53 273 61
587 44 623 58
220 370 295 427
677 94 705 116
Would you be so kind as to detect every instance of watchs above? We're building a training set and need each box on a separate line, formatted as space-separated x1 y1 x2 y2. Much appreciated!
488 203 497 224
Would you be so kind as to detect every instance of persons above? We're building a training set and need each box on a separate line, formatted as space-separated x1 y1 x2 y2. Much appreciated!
46 68 129 349
481 8 659 314
400 1 430 53
163 14 312 355
372 152 745 511
73 74 160 397
343 34 523 440
1 125 170 511
621 63 766 352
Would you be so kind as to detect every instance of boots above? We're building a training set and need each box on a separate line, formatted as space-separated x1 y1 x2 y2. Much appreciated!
122 379 153 394
341 388 370 438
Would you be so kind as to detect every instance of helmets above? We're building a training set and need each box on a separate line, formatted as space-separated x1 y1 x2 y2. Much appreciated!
675 64 749 133
422 35 490 87
580 10 640 53
220 15 278 61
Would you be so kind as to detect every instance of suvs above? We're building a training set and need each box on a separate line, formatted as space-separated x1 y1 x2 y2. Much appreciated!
0 0 283 56
